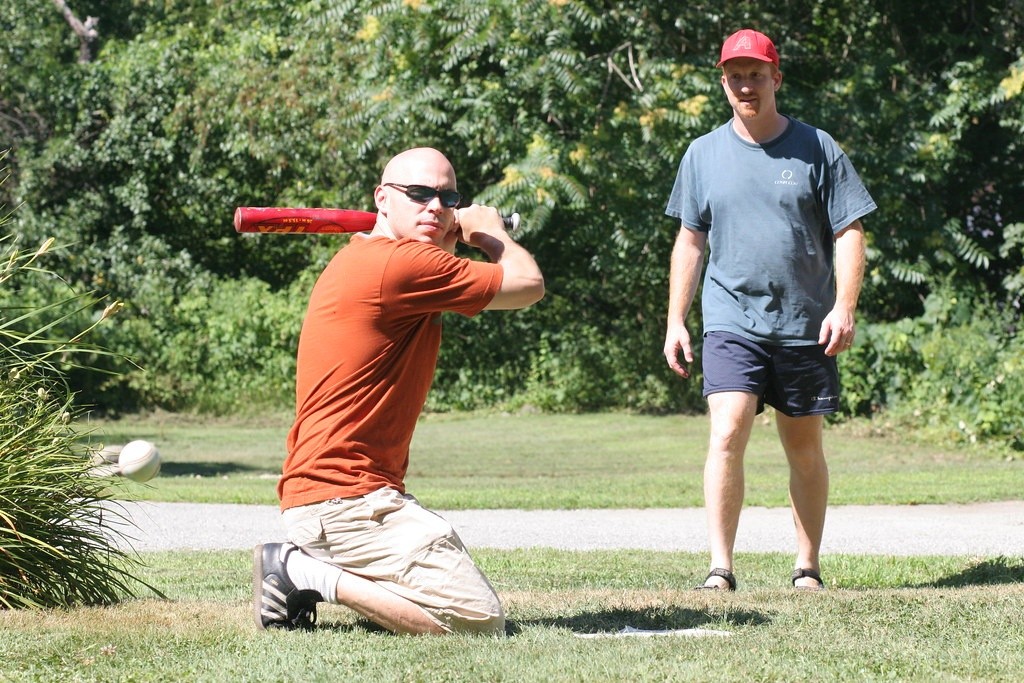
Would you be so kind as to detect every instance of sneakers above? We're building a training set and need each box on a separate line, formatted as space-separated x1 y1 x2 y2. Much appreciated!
254 541 324 632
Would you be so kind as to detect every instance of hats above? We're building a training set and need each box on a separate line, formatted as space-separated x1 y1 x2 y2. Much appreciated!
715 30 780 67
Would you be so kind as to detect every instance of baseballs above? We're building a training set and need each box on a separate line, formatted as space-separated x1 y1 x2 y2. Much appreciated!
118 439 162 482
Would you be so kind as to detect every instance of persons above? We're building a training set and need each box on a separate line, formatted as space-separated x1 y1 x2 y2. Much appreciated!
663 29 879 588
253 146 544 637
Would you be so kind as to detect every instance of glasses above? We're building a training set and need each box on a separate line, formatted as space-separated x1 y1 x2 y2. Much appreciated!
383 181 462 208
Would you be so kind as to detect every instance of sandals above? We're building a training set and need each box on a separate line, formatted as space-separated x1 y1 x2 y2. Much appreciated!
791 568 824 587
694 568 736 589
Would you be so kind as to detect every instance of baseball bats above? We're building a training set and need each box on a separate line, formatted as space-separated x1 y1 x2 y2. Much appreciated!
232 206 523 235
